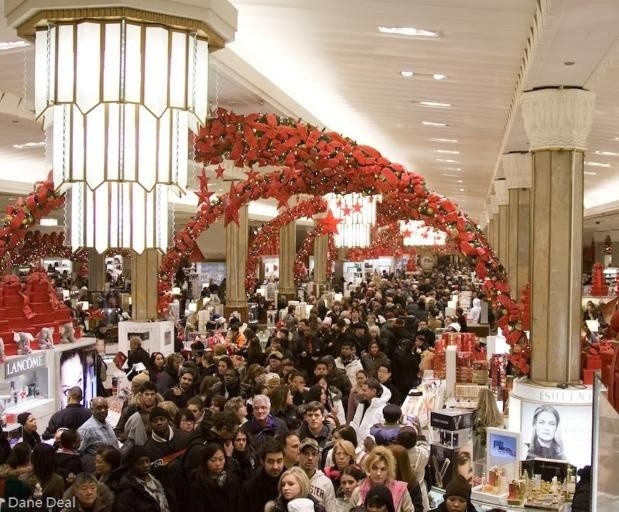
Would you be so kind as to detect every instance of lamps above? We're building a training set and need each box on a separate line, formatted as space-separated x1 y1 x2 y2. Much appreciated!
2 0 244 256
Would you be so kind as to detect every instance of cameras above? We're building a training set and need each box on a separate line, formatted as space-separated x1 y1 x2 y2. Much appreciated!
407 415 414 421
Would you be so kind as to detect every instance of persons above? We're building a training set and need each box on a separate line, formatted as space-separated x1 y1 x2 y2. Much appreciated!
0 261 493 511
582 300 606 327
526 403 567 460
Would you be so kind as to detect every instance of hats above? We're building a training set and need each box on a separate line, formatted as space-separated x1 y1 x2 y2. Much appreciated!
443 475 473 501
298 437 319 454
148 407 172 426
4 478 32 505
132 369 150 388
264 372 281 387
322 316 333 327
368 326 377 334
125 445 150 469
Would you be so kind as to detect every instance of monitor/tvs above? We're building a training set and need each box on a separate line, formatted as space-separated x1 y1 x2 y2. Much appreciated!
489 432 517 460
